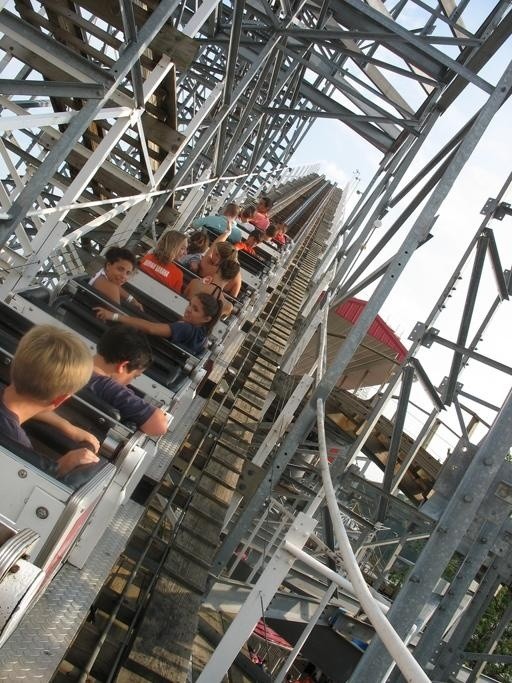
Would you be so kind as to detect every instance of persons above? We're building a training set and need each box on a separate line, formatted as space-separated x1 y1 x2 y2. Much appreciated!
139 197 287 320
91 291 224 355
87 245 144 312
1 320 103 479
75 321 168 436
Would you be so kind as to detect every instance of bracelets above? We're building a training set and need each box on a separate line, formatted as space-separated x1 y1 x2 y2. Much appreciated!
125 293 134 302
112 312 119 320
